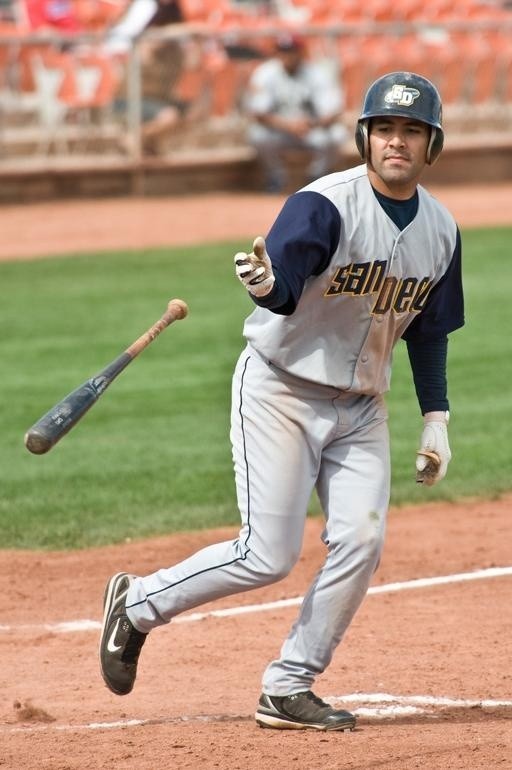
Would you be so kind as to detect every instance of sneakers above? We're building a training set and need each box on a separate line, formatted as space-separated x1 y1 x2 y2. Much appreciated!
100 572 147 695
256 692 356 731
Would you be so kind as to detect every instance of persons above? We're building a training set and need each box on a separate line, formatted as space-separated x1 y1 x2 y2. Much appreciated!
246 29 348 195
99 71 466 731
113 37 188 154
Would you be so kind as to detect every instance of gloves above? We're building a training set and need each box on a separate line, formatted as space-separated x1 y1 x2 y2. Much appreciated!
233 237 276 298
416 411 451 487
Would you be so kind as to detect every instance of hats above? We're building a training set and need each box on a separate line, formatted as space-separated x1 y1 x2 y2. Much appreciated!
274 34 299 50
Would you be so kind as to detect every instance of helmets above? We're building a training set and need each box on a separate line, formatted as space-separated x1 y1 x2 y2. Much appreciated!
359 71 442 130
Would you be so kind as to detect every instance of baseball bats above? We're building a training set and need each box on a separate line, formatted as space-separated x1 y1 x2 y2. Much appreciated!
24 299 187 455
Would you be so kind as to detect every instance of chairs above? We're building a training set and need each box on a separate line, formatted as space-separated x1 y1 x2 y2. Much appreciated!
1 1 512 176
